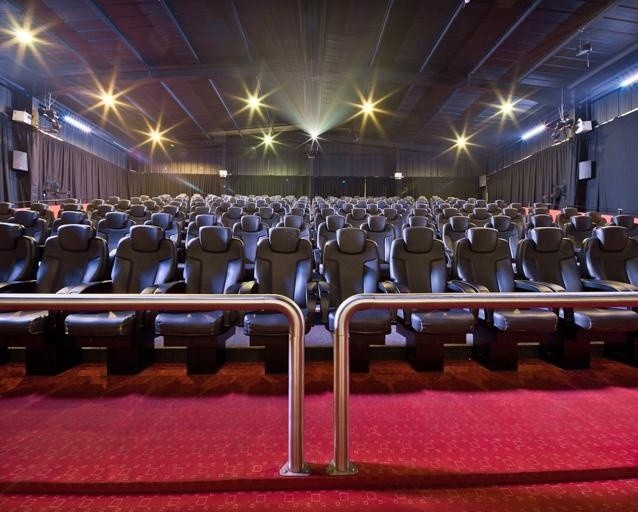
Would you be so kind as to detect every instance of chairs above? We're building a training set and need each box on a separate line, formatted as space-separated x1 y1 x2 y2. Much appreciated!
0 192 637 375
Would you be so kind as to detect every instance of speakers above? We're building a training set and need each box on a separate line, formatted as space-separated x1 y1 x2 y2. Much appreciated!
479 175 486 188
579 160 592 180
13 150 29 172
